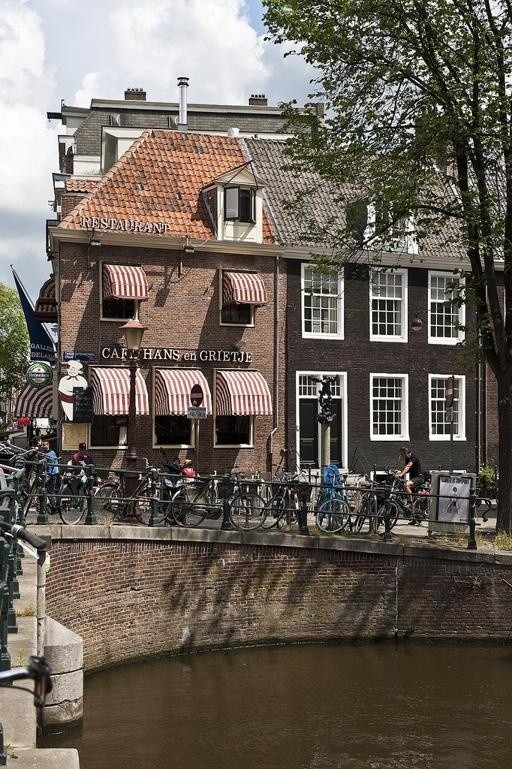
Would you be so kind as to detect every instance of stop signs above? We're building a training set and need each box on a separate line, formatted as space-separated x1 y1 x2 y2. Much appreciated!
190 384 203 407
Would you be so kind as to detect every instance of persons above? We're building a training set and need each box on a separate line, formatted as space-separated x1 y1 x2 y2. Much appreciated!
40 442 59 475
393 447 425 508
71 443 94 476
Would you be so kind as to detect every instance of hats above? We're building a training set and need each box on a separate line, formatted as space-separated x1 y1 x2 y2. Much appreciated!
79 442 86 450
